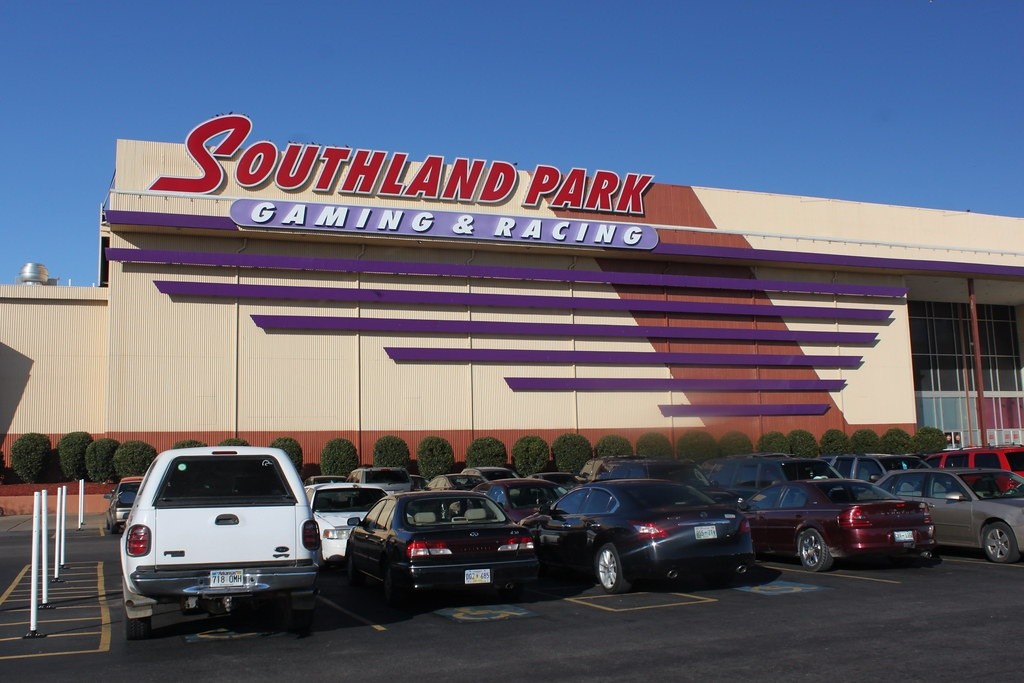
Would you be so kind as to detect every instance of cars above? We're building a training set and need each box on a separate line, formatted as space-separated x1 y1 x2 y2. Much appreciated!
526 472 587 491
594 460 723 504
575 455 648 482
304 483 389 562
471 478 568 524
345 490 541 603
857 468 1024 563
727 452 796 458
111 476 143 495
519 479 756 593
104 481 142 532
424 474 483 489
457 467 521 483
714 457 844 498
736 478 937 571
830 455 932 495
347 467 414 491
305 475 347 485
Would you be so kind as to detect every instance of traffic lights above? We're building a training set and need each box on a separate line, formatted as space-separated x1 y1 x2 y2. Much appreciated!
953 432 961 444
944 432 952 446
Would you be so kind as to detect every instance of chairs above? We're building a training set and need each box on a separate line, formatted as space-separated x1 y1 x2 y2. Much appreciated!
973 478 992 498
465 509 487 521
317 497 332 508
521 487 537 507
413 512 437 525
932 481 950 496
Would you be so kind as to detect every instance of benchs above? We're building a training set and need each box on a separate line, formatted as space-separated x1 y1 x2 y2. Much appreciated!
896 482 922 497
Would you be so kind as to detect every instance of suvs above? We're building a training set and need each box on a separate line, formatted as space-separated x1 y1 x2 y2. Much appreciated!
120 446 323 639
915 442 1024 492
